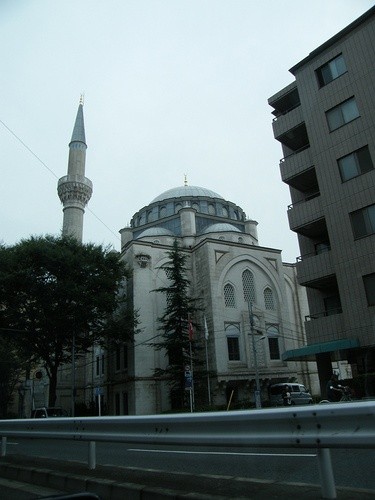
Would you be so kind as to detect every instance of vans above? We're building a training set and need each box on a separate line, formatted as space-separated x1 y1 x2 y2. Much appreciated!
270 383 314 405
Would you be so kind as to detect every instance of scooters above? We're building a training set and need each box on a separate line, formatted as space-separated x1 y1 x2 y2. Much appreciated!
317 385 354 404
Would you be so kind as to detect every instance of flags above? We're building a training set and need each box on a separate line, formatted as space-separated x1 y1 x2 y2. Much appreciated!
204 316 209 340
189 322 192 341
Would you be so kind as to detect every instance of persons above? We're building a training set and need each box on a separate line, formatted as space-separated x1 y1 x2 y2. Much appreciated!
281 384 292 406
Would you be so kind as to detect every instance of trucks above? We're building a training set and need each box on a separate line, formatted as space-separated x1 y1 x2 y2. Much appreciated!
21 407 70 418
